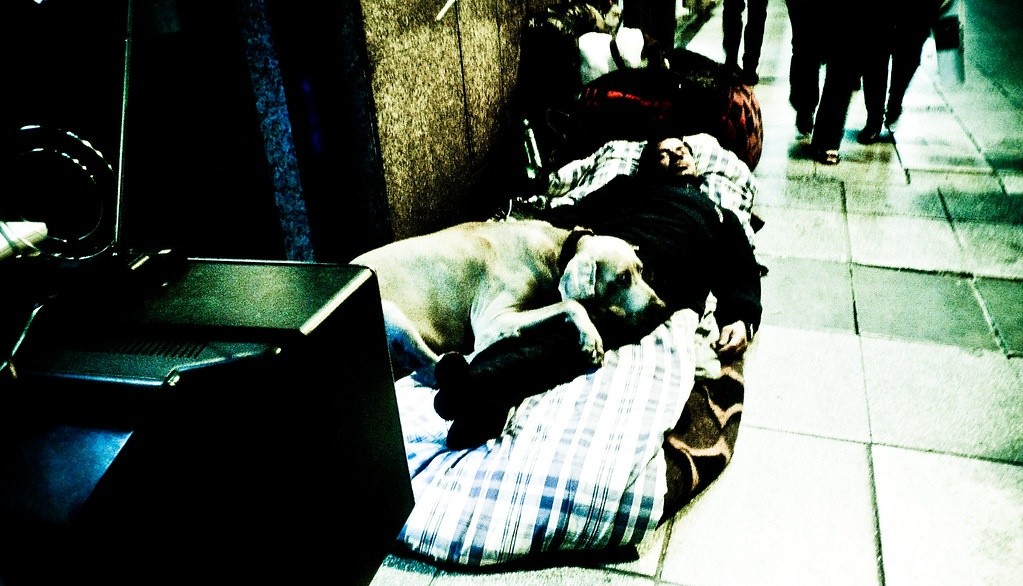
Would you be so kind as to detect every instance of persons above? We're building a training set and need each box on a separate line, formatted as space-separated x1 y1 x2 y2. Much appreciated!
787 0 944 165
722 1 768 85
433 131 764 454
621 0 677 62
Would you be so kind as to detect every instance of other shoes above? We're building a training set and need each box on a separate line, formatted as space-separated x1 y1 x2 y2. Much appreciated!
812 139 839 164
884 104 900 135
857 120 884 144
743 65 760 87
796 110 813 136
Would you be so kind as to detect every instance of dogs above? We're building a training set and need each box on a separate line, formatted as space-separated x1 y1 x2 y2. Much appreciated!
351 213 665 384
